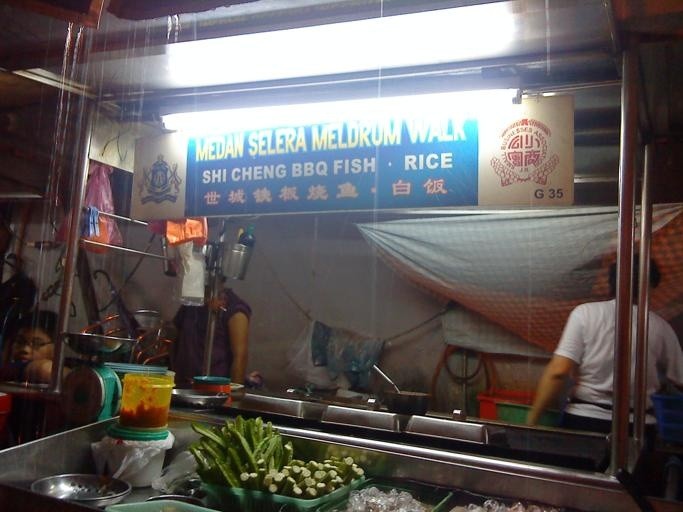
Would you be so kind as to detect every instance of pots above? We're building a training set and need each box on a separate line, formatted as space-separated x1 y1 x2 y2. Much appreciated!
372 362 432 416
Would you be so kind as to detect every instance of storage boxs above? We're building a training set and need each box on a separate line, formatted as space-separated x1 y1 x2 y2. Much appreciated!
497 402 563 427
478 389 542 425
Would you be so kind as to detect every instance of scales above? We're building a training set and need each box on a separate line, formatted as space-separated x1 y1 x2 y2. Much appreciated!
60 331 139 429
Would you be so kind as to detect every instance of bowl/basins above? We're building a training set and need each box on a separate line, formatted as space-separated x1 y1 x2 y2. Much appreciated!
60 330 138 358
169 388 229 409
135 309 161 327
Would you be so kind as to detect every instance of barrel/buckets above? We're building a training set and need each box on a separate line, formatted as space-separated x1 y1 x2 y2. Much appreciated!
221 220 253 282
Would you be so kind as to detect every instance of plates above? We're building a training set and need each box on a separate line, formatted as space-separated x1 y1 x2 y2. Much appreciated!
30 473 132 507
192 375 232 394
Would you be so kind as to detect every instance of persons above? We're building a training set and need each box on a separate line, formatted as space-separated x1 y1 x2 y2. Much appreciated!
1 310 73 385
523 249 683 479
171 274 250 392
